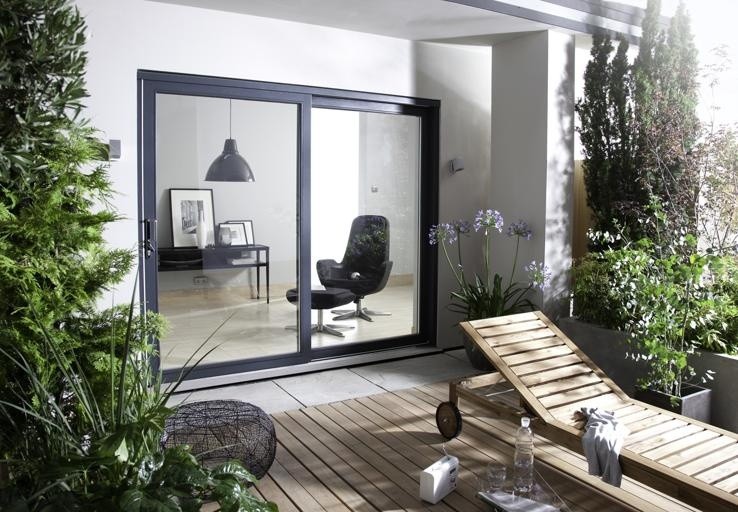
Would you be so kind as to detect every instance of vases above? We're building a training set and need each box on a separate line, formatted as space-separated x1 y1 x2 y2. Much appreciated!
218 227 231 246
464 333 497 372
196 210 207 248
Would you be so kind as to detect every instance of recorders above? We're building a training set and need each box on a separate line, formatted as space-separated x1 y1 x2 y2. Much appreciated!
420 454 459 504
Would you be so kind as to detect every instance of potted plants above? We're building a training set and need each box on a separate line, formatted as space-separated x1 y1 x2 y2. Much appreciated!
586 194 738 424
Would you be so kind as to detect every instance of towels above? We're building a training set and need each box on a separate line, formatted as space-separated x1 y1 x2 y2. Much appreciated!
582 408 628 488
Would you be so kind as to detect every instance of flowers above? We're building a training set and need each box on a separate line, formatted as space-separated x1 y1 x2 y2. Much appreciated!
428 208 552 334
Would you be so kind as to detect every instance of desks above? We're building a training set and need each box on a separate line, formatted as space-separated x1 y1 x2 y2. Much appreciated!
158 245 269 304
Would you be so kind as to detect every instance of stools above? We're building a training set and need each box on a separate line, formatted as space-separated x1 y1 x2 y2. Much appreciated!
286 288 356 337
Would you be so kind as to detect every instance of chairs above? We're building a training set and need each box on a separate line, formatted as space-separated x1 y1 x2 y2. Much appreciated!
450 311 738 512
319 215 393 322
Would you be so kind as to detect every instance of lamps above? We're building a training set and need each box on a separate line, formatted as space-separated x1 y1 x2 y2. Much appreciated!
204 99 256 182
451 158 464 173
109 140 121 161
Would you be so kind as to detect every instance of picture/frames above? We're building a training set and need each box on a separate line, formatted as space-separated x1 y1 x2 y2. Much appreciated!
169 189 216 249
219 223 248 247
228 220 254 246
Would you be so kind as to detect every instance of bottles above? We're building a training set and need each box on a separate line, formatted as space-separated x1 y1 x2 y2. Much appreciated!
513 417 534 492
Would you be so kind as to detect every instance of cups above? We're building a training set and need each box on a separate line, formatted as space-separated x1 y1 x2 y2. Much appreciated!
487 461 506 490
